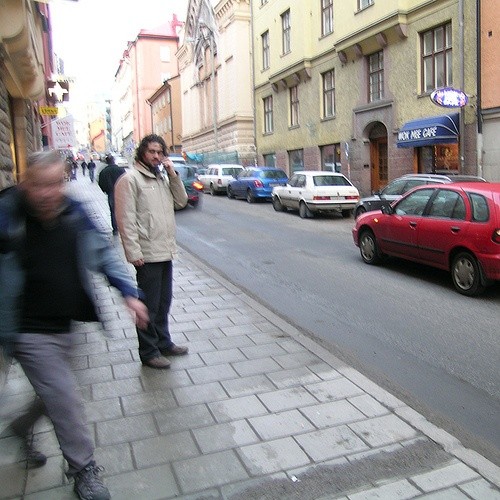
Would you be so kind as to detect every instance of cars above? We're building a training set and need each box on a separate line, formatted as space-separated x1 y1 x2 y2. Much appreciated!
352 181 500 296
159 165 200 207
90 152 129 168
269 170 361 219
160 156 187 165
353 172 489 224
227 165 291 203
199 163 245 195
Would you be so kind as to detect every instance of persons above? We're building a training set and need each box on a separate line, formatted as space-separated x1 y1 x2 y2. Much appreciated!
114 134 189 367
0 150 150 500
81 160 88 175
87 159 96 183
98 155 126 235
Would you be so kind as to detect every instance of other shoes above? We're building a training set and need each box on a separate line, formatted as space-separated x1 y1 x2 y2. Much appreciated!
113 230 118 236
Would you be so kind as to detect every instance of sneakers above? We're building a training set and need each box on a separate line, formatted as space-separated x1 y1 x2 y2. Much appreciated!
141 355 171 368
2 430 47 466
160 342 188 354
74 460 110 500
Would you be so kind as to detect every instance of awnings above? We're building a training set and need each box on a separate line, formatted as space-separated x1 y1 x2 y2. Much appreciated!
397 112 459 148
